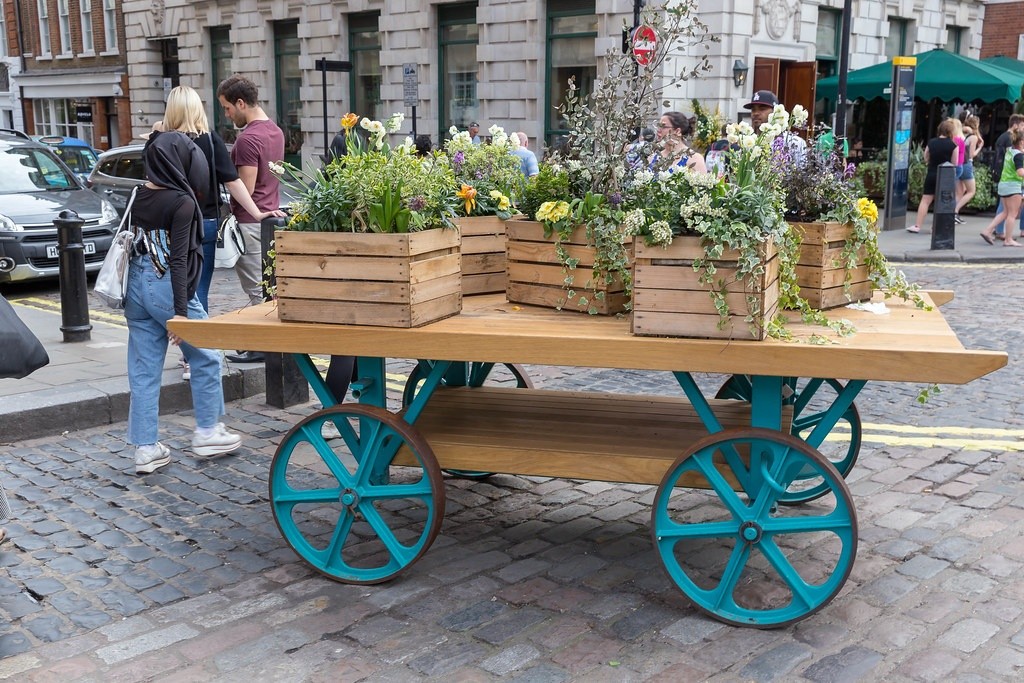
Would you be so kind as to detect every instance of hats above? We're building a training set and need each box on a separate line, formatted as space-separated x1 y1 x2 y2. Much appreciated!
470 122 479 127
743 90 778 109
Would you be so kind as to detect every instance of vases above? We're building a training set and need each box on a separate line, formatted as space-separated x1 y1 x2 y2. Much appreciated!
506 218 632 315
630 235 780 341
788 221 873 309
450 209 528 295
274 225 462 328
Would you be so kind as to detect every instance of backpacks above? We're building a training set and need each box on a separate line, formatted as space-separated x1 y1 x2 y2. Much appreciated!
705 141 738 183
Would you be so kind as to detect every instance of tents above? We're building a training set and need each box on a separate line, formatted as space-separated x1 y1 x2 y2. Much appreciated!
815 46 1024 104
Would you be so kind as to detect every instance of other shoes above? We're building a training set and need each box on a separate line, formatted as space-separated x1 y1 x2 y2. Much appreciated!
906 225 920 233
1003 242 1022 247
980 232 993 244
955 213 967 224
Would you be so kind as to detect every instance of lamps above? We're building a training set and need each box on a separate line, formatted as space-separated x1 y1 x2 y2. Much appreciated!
733 60 749 86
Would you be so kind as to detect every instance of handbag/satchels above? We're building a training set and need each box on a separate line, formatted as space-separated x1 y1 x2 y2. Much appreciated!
93 230 135 310
213 213 248 269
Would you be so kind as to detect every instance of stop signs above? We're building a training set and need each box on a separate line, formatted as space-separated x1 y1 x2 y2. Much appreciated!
633 25 657 67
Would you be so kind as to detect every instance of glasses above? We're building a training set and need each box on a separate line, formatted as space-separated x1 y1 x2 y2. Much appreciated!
657 124 678 130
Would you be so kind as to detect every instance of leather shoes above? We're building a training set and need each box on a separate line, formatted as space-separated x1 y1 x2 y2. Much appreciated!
225 350 266 363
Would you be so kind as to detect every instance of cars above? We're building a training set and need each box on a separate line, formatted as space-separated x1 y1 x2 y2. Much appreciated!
86 141 313 231
0 129 125 284
28 134 99 188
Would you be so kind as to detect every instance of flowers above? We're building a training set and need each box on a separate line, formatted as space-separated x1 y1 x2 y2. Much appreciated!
238 0 942 403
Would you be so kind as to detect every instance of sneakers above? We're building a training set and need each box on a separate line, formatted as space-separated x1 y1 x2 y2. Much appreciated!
190 421 242 456
135 442 171 473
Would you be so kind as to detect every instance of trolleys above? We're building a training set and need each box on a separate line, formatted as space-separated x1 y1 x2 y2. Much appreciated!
165 288 1007 632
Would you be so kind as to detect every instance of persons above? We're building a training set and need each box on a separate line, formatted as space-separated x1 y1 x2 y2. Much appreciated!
931 117 964 230
980 114 1024 247
743 90 779 136
142 85 288 380
300 125 372 438
501 132 540 183
906 121 959 234
618 112 707 185
215 74 284 362
123 134 242 474
465 122 481 146
954 110 984 224
848 138 862 157
553 120 572 150
704 123 744 184
